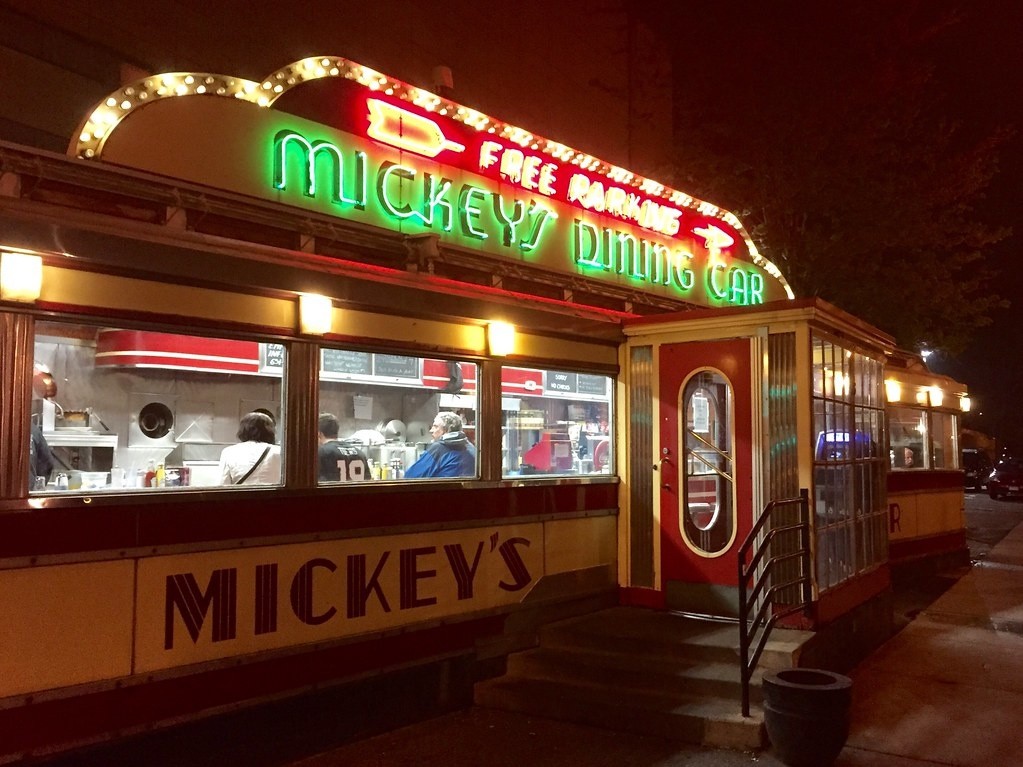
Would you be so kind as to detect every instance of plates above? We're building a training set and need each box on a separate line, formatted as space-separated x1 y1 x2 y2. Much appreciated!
44 426 99 434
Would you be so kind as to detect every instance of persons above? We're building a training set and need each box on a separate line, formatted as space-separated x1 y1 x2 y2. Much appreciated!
26 417 54 499
402 410 478 479
315 409 373 486
903 444 915 469
217 410 282 484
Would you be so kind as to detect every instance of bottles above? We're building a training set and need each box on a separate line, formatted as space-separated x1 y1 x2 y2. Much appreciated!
145 460 157 487
110 464 125 490
156 465 166 488
135 468 146 488
367 458 405 480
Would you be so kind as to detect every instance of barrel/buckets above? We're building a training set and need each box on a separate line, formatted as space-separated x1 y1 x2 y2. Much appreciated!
81 471 109 492
761 666 855 763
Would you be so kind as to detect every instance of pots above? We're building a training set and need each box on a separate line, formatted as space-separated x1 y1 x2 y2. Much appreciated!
32 360 111 431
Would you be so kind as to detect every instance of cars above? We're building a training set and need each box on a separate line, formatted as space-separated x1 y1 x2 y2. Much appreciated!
961 448 992 492
988 459 1023 500
